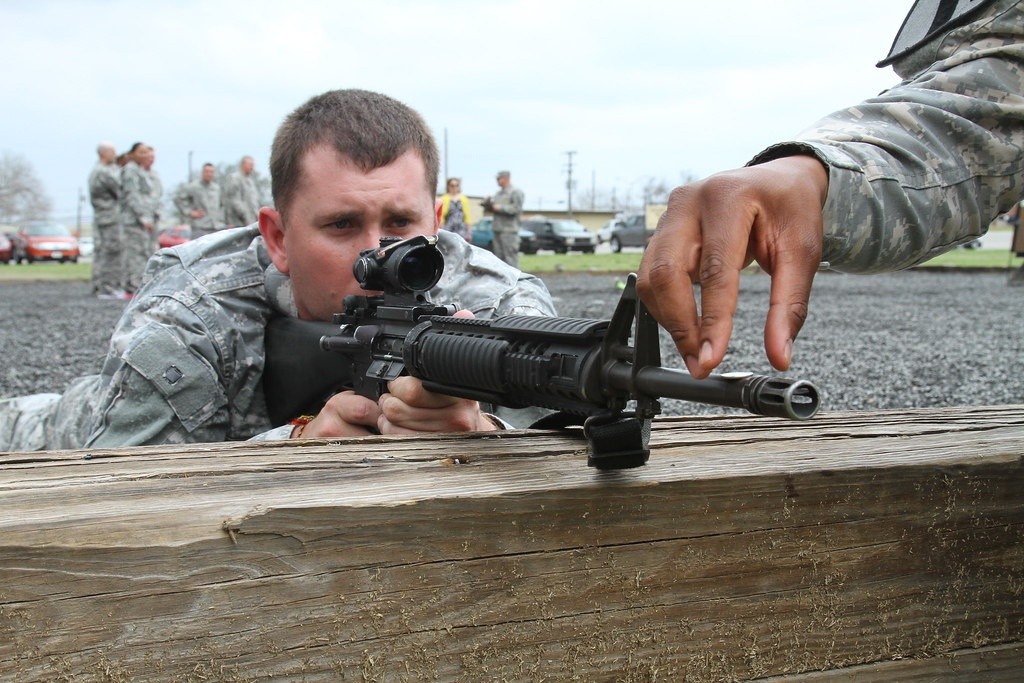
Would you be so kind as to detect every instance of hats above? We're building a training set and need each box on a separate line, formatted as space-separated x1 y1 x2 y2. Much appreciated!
495 172 510 179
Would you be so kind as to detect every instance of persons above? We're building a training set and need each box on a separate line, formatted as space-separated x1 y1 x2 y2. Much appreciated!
439 179 472 244
91 140 164 297
174 157 269 241
0 90 560 451
482 170 525 268
635 0 1024 380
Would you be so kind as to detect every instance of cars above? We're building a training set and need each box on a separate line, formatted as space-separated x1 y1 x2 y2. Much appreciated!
597 220 627 243
79 237 95 256
159 224 190 246
18 219 81 264
0 230 14 263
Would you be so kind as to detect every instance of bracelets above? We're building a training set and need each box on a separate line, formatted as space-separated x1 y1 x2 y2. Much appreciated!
289 414 314 437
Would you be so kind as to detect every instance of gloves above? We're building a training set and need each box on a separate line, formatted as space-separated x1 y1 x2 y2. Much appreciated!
479 194 493 211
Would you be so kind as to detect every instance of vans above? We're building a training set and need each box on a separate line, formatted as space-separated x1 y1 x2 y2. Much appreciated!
528 219 597 255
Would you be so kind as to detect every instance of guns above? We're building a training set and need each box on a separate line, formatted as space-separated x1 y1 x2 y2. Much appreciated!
262 234 821 435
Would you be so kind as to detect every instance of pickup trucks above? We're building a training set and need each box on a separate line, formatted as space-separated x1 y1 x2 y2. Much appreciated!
576 235 579 236
610 214 656 252
471 218 536 256
